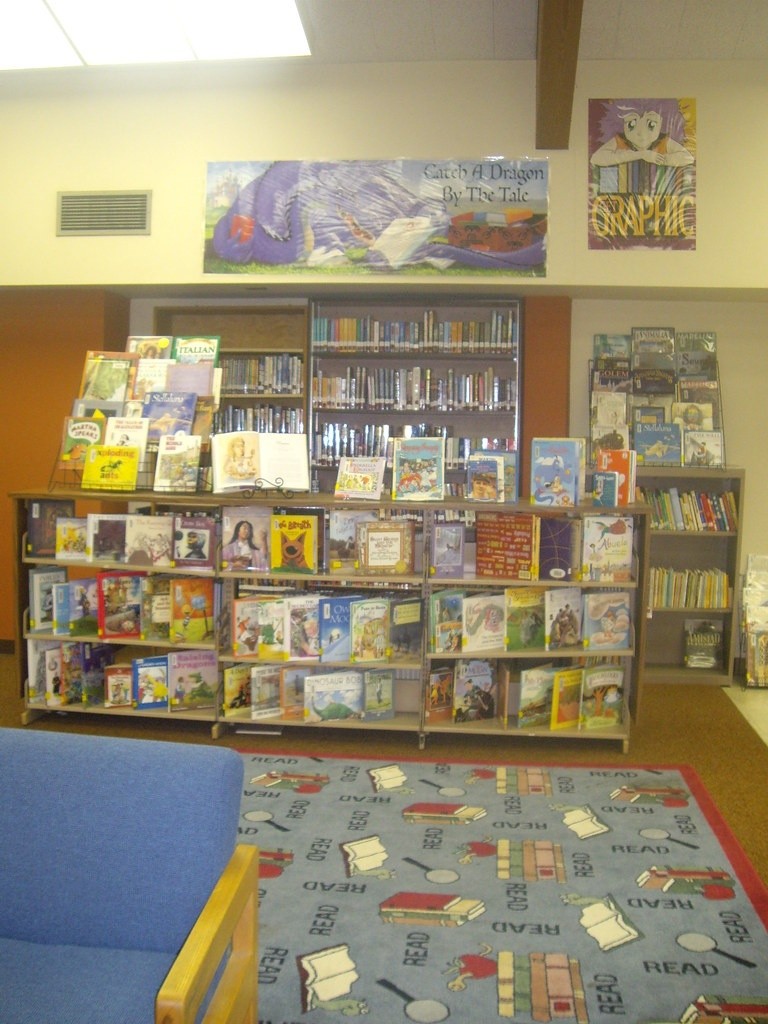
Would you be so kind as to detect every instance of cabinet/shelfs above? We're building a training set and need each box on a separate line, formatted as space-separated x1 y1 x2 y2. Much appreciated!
627 466 748 688
149 302 523 499
5 491 652 754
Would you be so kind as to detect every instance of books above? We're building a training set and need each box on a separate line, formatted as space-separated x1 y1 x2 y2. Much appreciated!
25 311 768 737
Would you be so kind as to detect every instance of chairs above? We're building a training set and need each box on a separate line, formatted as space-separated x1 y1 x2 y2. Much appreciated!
0 727 260 1024
